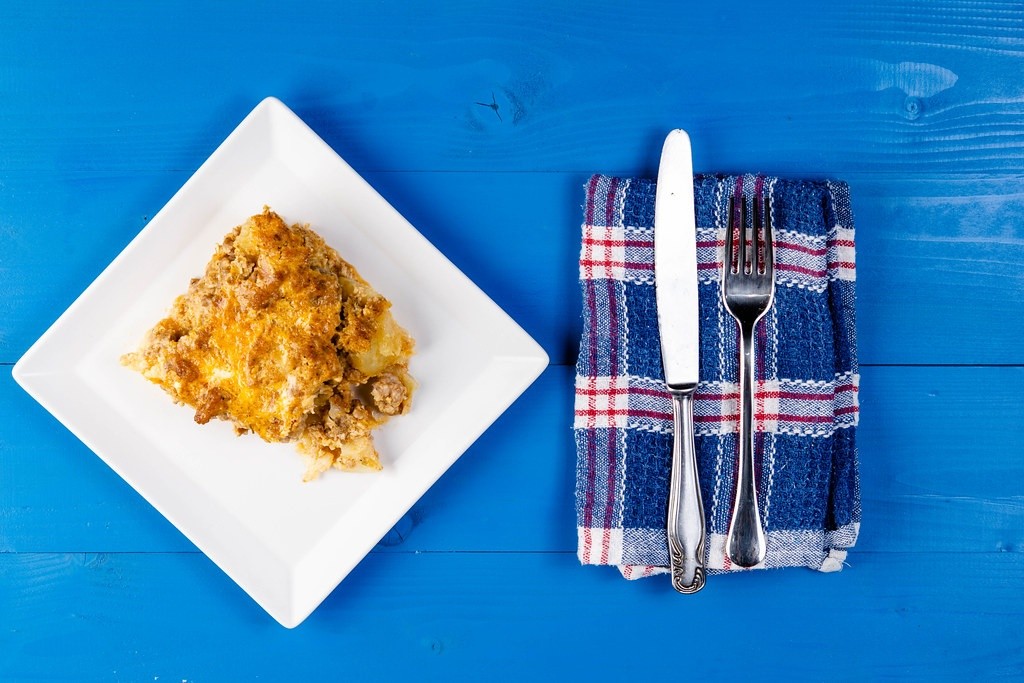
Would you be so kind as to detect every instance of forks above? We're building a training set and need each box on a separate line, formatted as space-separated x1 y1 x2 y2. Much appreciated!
720 195 777 567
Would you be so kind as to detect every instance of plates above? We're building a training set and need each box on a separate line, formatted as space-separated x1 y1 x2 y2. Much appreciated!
10 95 552 630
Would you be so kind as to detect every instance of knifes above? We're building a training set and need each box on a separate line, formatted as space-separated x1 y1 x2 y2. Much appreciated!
653 127 706 595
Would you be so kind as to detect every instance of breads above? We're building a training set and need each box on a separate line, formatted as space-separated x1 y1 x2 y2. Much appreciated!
119 206 415 479
